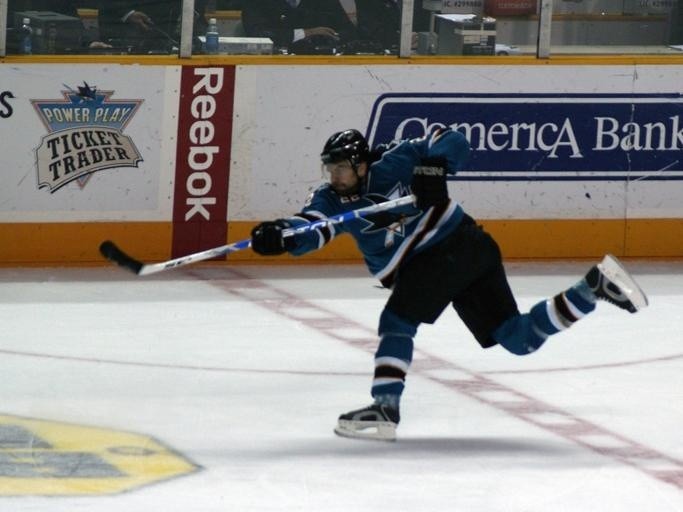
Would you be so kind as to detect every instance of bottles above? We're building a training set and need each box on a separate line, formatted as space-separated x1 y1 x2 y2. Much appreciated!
204 17 220 57
14 18 35 56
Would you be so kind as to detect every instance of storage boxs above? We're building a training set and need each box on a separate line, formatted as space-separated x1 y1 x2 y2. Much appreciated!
11 11 82 54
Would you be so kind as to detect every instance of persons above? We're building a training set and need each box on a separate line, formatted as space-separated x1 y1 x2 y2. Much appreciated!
248 127 640 425
240 0 432 50
5 0 208 53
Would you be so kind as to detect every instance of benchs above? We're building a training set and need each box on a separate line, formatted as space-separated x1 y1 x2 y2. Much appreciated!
63 7 683 56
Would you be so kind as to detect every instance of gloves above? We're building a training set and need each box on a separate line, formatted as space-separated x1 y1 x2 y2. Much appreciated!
411 156 451 210
250 219 292 256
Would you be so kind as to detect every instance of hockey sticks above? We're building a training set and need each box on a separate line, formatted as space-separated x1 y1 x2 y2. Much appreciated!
99 194 417 277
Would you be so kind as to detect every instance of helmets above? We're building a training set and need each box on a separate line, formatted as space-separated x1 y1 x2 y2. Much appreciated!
321 129 370 168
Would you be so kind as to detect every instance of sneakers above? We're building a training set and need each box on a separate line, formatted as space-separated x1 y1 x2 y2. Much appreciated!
338 404 400 424
586 265 637 313
